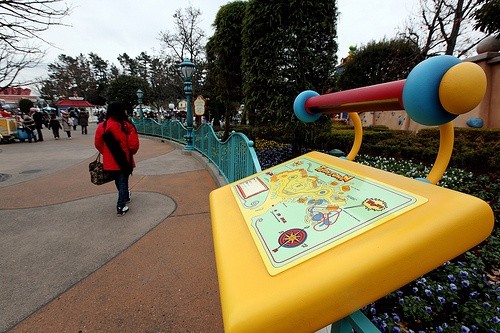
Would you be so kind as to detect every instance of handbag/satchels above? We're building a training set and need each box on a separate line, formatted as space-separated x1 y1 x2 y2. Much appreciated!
102 121 133 176
89 152 115 185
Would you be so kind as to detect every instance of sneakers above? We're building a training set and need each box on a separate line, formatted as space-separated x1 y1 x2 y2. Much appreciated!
56 137 58 140
129 191 131 199
117 205 129 216
68 137 71 139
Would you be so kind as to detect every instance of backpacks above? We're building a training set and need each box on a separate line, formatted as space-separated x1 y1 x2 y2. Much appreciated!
63 117 74 126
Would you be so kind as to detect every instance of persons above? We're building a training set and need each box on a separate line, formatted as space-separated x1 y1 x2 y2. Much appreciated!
0 106 210 140
95 103 139 216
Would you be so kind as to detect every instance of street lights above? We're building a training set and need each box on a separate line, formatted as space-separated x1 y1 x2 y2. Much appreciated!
136 89 144 126
177 57 195 149
